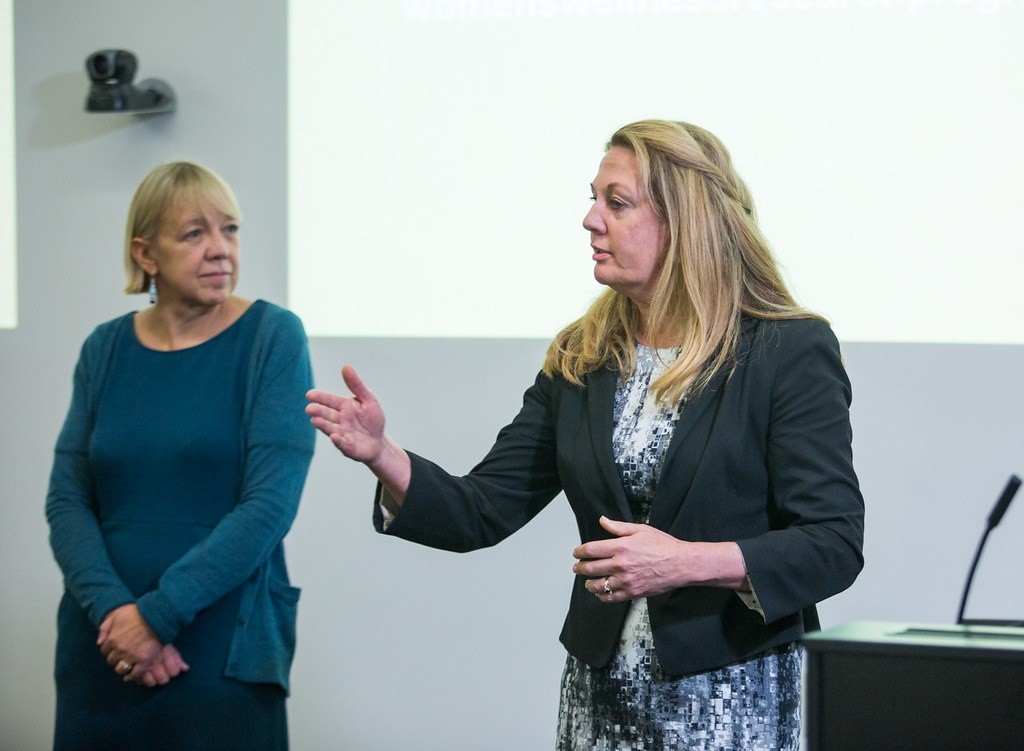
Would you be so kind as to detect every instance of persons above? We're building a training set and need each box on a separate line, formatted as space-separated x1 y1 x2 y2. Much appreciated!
45 163 318 751
305 121 866 751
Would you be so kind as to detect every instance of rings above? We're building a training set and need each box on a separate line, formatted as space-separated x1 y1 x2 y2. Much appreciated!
603 576 614 595
120 660 133 670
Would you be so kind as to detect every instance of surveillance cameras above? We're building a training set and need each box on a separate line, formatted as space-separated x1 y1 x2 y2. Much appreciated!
84 48 162 112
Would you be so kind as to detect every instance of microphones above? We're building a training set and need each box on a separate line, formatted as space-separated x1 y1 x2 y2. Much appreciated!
957 474 1021 623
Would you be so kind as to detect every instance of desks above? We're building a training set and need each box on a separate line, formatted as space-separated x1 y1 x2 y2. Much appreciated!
801 620 1024 751
